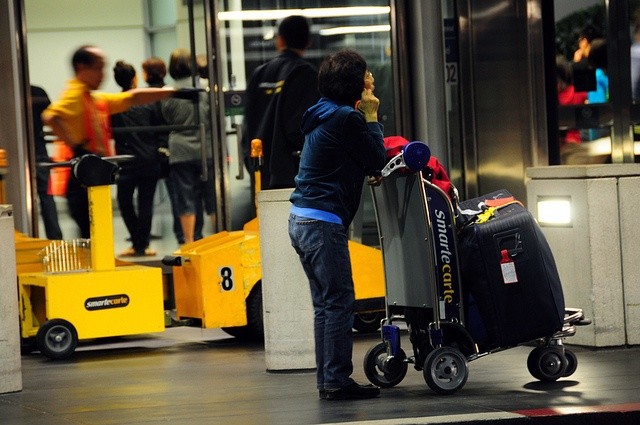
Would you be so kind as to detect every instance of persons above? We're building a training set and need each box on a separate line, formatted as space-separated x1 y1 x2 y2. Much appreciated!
30 82 65 240
161 46 222 245
109 60 156 257
132 58 187 249
287 50 387 402
239 15 324 191
41 45 208 240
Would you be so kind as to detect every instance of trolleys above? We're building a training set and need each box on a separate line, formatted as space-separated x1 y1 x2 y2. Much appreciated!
363 141 592 395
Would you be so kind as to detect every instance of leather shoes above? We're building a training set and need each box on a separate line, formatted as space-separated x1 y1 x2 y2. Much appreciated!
318 382 375 401
322 381 385 402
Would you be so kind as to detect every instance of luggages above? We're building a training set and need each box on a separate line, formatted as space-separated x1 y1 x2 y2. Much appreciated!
454 187 567 350
